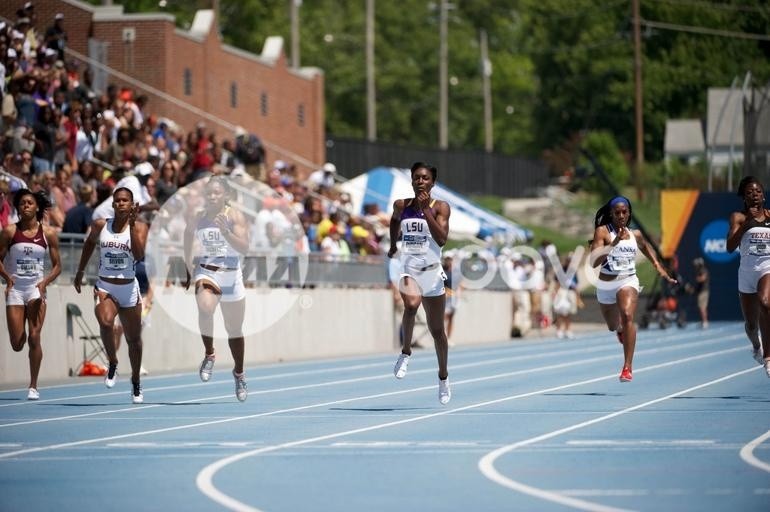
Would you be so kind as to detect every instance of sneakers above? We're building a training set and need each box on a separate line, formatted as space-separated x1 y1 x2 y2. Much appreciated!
200 349 215 382
131 377 143 404
762 357 770 376
620 367 633 381
394 353 411 379
233 369 248 401
106 363 117 388
617 332 624 344
752 347 763 364
28 387 39 399
438 371 451 405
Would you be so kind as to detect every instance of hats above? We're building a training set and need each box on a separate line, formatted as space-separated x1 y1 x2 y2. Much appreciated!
134 162 154 176
330 225 338 233
11 154 24 163
351 226 369 238
324 163 336 173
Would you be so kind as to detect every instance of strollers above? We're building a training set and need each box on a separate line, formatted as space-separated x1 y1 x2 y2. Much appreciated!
637 259 689 330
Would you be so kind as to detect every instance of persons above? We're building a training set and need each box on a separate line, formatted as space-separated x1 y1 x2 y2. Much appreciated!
727 174 770 379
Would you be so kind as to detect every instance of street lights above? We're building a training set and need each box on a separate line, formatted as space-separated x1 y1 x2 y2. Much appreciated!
318 32 378 138
